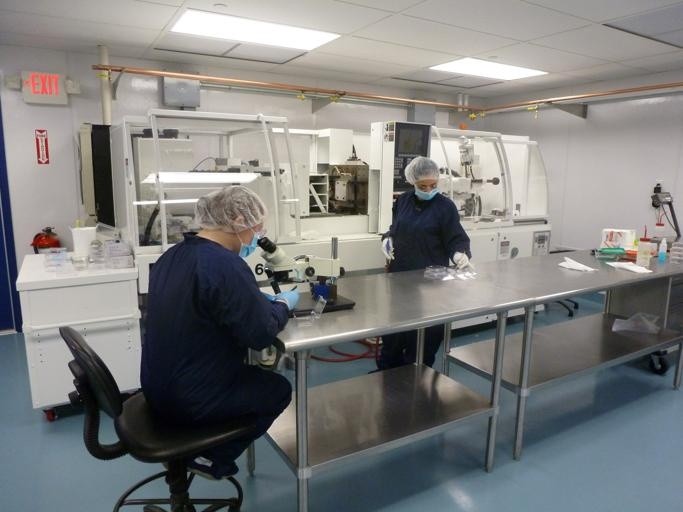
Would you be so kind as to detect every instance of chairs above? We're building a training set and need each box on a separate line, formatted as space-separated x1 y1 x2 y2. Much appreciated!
163 454 239 479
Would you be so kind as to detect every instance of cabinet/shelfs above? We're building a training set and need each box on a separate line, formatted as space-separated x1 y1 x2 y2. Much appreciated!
636 238 651 267
658 238 667 263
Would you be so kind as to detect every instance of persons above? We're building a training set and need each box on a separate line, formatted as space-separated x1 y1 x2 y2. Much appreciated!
366 151 475 375
138 184 300 482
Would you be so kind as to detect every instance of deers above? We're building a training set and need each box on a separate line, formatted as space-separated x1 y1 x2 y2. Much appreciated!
414 183 440 201
237 227 260 258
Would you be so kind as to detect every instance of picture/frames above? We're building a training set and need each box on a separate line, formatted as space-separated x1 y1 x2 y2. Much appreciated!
258 244 683 510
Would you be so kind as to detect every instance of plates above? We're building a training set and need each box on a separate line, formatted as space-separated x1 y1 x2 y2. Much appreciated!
14 248 144 422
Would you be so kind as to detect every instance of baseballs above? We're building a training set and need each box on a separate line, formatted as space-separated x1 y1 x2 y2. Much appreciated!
32 227 61 253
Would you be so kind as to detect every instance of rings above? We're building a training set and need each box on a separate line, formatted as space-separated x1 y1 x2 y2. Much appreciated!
291 286 297 291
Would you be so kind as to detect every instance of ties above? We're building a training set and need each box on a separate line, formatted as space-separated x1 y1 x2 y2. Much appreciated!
395 123 429 158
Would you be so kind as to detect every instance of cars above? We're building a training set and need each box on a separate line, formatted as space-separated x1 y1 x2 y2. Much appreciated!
72 226 97 259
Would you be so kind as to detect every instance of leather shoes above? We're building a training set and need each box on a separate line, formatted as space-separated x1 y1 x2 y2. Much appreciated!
262 288 299 312
452 252 470 272
382 237 395 260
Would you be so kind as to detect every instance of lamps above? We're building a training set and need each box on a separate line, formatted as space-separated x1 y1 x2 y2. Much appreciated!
59 324 257 512
